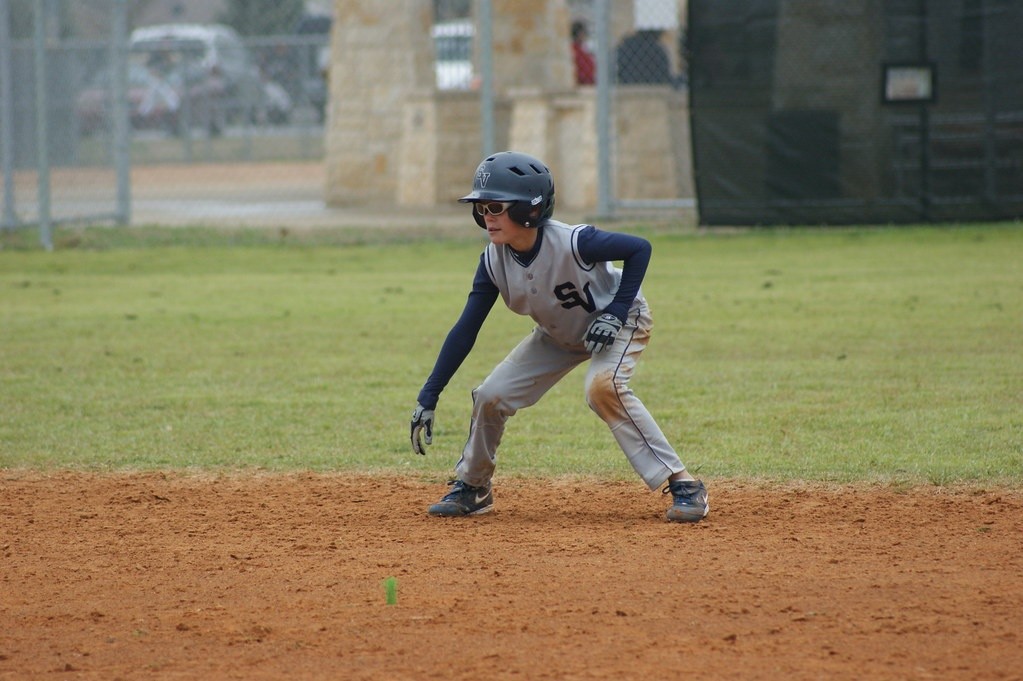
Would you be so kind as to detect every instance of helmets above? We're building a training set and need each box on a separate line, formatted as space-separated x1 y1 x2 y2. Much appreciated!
457 151 556 228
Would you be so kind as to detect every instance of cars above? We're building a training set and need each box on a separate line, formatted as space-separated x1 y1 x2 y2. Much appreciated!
72 54 223 139
248 47 330 127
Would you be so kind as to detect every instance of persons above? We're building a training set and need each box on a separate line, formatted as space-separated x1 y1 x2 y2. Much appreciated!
572 21 596 85
410 152 710 522
615 29 676 84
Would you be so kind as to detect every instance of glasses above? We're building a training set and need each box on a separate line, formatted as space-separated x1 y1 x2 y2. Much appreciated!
475 202 514 216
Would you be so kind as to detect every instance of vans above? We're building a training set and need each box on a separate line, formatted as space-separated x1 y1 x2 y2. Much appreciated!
433 22 474 92
124 23 256 126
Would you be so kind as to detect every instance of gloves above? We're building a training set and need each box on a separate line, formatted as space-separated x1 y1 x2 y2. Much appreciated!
410 403 434 456
581 313 623 354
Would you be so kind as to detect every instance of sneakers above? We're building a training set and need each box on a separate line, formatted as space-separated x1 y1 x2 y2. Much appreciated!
428 480 495 515
666 479 710 521
